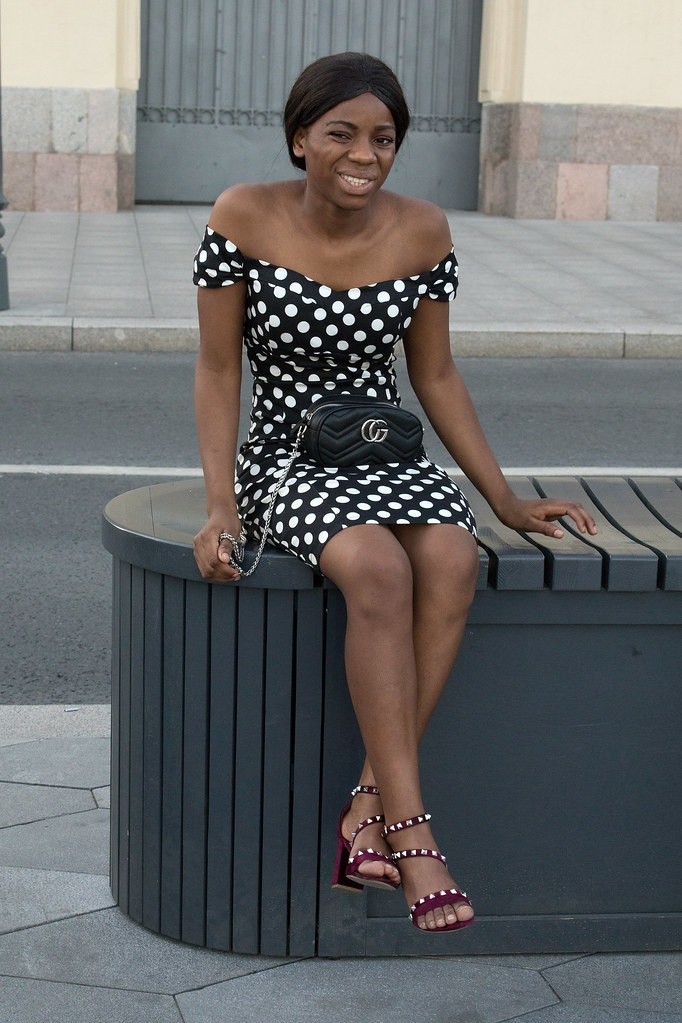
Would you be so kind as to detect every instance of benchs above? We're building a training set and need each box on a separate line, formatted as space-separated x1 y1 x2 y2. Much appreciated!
101 473 681 961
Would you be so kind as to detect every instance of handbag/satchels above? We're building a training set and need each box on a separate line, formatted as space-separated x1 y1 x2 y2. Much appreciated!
297 393 425 469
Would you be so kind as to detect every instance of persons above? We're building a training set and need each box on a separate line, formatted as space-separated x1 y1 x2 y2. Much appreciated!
191 52 598 931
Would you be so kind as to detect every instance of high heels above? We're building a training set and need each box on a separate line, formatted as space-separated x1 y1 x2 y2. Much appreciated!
330 785 401 893
379 813 475 934
